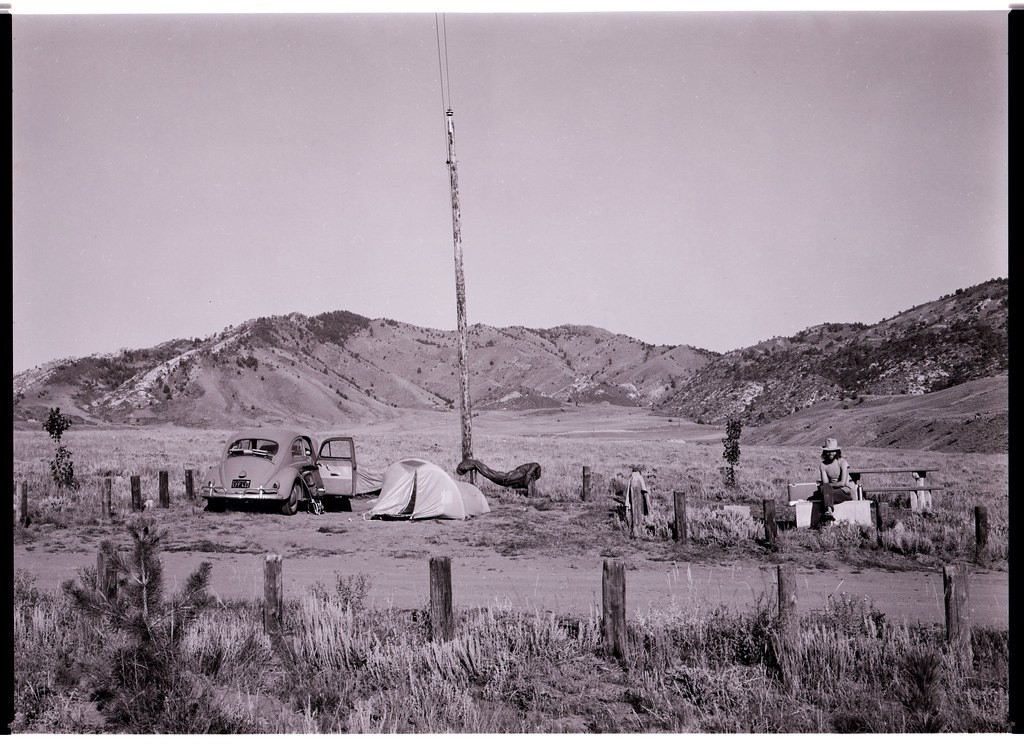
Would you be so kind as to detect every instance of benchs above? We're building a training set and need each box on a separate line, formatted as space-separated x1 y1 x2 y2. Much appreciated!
795 499 872 528
862 485 944 509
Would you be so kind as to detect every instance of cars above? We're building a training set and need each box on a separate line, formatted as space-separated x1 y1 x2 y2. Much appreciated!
199 428 358 516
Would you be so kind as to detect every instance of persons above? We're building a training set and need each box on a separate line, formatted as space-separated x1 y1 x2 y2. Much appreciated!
815 438 852 520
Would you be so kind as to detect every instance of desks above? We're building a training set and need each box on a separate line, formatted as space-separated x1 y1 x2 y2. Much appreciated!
849 466 941 511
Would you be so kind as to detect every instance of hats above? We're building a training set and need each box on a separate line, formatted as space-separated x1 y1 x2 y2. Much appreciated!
821 438 841 450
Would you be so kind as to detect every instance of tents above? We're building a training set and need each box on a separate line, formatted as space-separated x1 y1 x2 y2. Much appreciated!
362 458 490 522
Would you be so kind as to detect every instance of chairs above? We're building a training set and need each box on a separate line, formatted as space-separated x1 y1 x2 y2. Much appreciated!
257 445 276 459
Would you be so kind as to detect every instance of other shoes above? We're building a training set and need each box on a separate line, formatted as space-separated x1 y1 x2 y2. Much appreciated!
825 511 836 521
